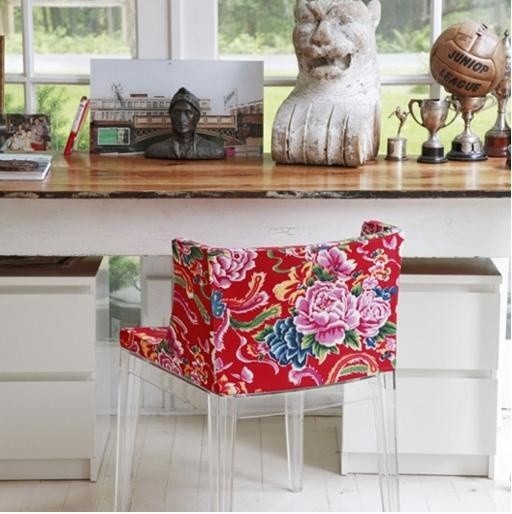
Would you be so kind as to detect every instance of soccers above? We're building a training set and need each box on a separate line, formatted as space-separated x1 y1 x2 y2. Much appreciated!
430 22 506 96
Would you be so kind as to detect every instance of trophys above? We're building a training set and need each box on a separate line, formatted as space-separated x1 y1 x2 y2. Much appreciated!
484 26 510 158
428 21 506 162
382 106 410 161
407 97 459 166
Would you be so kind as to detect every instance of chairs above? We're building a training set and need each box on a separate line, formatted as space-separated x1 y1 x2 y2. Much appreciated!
114 220 406 512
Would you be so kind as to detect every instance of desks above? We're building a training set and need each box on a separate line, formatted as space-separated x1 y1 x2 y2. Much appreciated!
0 150 512 483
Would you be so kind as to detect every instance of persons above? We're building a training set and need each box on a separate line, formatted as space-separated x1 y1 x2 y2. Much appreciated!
0 117 45 151
140 88 226 160
116 130 129 144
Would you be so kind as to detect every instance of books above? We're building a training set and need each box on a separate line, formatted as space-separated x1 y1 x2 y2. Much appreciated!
64 96 90 155
